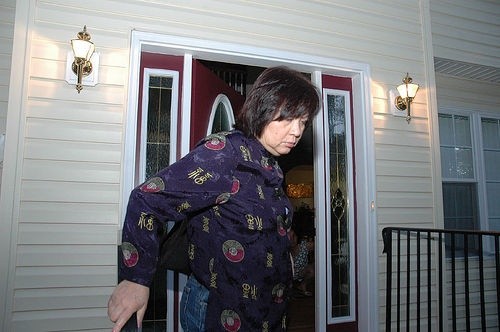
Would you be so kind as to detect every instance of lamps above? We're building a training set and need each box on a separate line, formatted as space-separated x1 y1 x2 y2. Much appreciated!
395 72 419 125
66 25 99 93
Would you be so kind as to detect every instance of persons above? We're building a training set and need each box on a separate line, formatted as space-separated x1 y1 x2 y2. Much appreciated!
107 65 321 331
